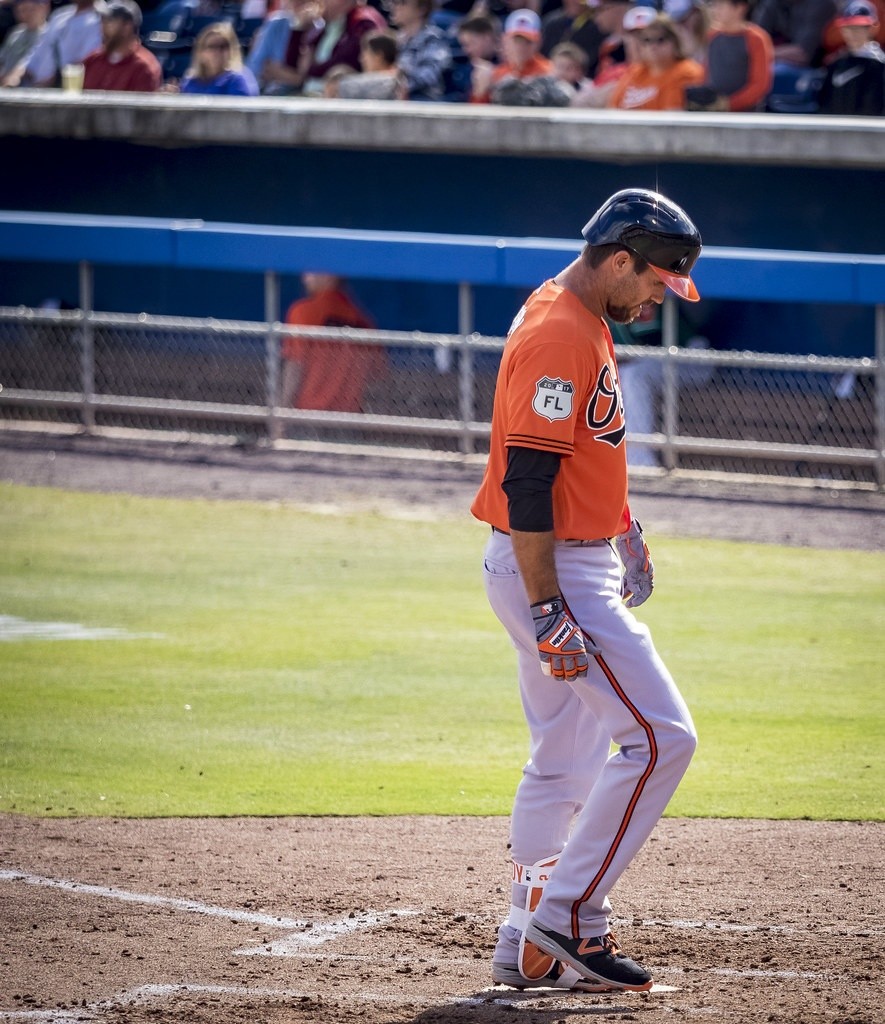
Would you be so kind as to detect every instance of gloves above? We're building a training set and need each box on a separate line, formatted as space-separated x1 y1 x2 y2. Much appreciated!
615 518 655 609
530 595 602 683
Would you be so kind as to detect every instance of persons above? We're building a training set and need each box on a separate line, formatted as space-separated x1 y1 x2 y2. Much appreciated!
0 0 885 117
606 296 717 466
279 273 387 445
470 186 703 992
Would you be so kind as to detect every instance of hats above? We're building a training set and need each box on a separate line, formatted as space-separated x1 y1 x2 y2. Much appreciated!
503 9 540 44
835 0 877 27
93 0 143 29
623 7 659 32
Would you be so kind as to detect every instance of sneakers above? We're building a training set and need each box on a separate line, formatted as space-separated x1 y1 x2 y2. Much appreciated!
489 924 622 991
526 914 656 994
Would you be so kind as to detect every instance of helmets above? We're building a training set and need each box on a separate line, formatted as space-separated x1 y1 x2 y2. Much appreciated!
581 187 706 303
